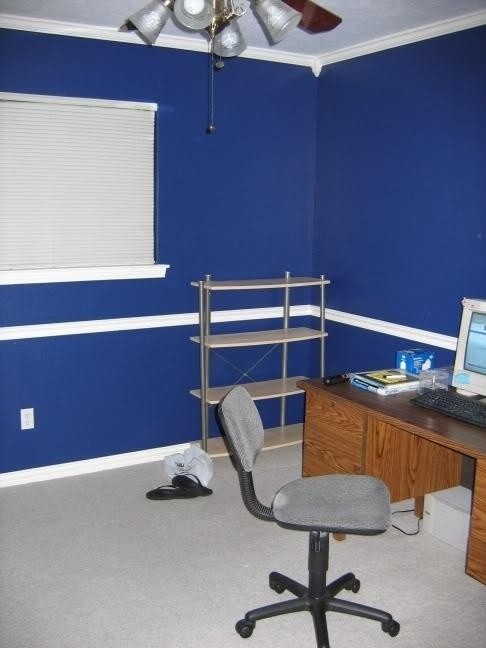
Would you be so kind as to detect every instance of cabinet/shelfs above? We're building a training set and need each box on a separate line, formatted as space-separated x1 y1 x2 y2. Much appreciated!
190 270 331 457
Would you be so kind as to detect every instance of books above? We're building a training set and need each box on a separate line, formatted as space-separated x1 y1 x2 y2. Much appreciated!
349 368 421 389
351 377 420 395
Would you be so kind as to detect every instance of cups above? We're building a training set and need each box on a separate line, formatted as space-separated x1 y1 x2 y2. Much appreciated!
419 369 450 397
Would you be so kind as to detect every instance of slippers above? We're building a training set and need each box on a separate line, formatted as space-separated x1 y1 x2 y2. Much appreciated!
172 474 213 496
146 485 196 500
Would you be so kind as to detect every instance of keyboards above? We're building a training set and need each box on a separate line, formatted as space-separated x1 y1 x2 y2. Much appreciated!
409 388 485 428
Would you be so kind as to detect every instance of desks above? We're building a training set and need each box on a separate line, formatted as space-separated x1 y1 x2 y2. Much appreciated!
296 365 486 586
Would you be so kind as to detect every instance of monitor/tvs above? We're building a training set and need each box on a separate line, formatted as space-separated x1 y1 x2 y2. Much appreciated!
452 297 486 403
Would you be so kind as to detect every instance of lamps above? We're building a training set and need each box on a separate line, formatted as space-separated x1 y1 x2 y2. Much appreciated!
127 0 303 59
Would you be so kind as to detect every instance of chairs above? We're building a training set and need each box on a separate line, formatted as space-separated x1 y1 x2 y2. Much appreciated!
213 385 399 648
